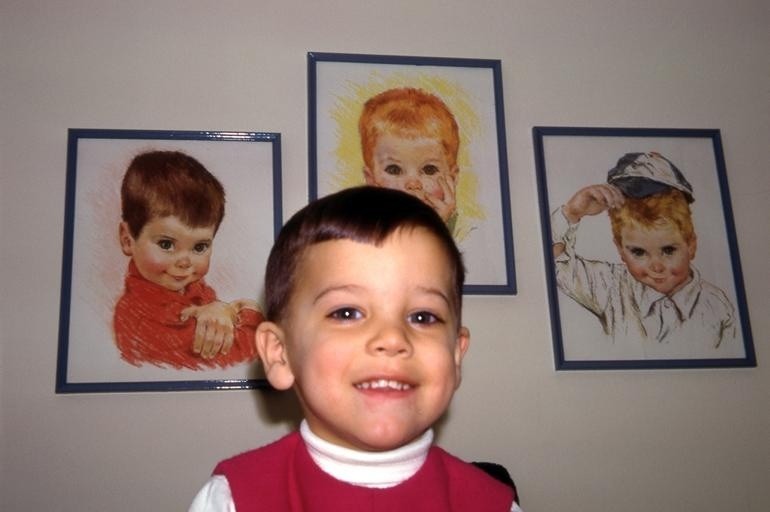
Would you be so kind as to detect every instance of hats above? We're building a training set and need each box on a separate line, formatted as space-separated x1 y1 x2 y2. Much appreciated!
606 150 696 203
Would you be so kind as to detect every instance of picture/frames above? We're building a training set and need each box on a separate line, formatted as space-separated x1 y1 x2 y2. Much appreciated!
56 126 291 395
305 52 520 298
531 123 760 374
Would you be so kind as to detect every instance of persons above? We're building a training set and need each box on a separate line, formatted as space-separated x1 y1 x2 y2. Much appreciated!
550 153 736 348
360 88 460 237
114 151 264 370
189 186 523 512
